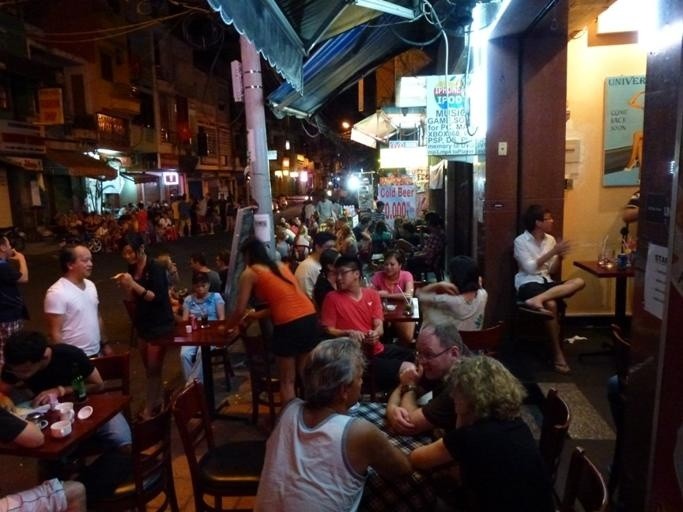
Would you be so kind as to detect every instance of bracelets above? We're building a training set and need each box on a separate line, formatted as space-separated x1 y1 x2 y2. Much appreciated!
140 289 147 297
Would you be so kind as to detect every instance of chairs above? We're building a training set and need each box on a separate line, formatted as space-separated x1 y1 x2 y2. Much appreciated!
171 379 282 508
515 259 566 362
552 440 611 510
86 351 133 399
99 410 179 508
518 388 575 484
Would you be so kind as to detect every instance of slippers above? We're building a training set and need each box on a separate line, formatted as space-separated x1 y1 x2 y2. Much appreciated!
554 363 572 375
518 305 554 320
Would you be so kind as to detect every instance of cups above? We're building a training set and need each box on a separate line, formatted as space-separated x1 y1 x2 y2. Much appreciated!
54 402 75 424
50 420 71 439
25 412 44 427
597 248 615 271
185 326 191 332
378 291 387 315
401 294 413 316
198 305 209 327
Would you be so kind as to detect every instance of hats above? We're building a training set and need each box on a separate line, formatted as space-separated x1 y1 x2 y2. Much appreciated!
449 255 479 290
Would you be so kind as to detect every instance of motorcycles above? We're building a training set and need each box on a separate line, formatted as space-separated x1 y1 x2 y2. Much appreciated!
64 217 108 255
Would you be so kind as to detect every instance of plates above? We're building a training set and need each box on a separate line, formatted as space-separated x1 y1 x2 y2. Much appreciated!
39 419 49 429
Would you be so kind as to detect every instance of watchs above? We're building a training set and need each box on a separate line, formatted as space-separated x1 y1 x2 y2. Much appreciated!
400 384 417 396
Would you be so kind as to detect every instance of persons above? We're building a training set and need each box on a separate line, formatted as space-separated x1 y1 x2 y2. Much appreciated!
54 192 234 249
409 354 543 511
252 334 414 512
386 318 475 436
176 250 230 386
622 189 640 223
2 236 31 323
0 407 88 511
0 326 135 460
510 200 586 373
219 187 489 408
44 243 114 361
115 231 175 420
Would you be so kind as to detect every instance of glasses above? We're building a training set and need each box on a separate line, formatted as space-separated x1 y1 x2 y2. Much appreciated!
415 346 452 360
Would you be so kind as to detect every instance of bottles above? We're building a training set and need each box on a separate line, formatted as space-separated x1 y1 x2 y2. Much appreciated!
187 308 198 330
70 362 89 404
